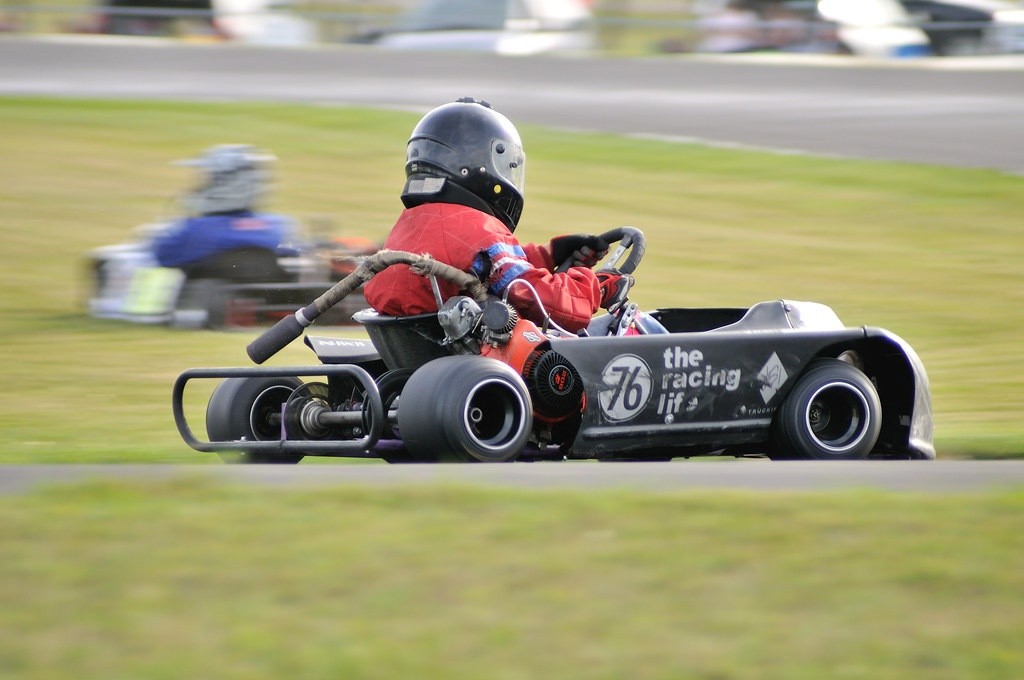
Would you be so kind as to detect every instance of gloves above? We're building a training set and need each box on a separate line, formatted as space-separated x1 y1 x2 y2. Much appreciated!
594 267 635 309
550 234 609 272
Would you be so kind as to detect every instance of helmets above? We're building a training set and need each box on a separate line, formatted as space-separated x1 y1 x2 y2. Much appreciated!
171 142 280 212
401 97 526 234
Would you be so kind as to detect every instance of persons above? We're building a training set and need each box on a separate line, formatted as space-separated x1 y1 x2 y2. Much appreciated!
153 144 294 262
363 100 671 338
